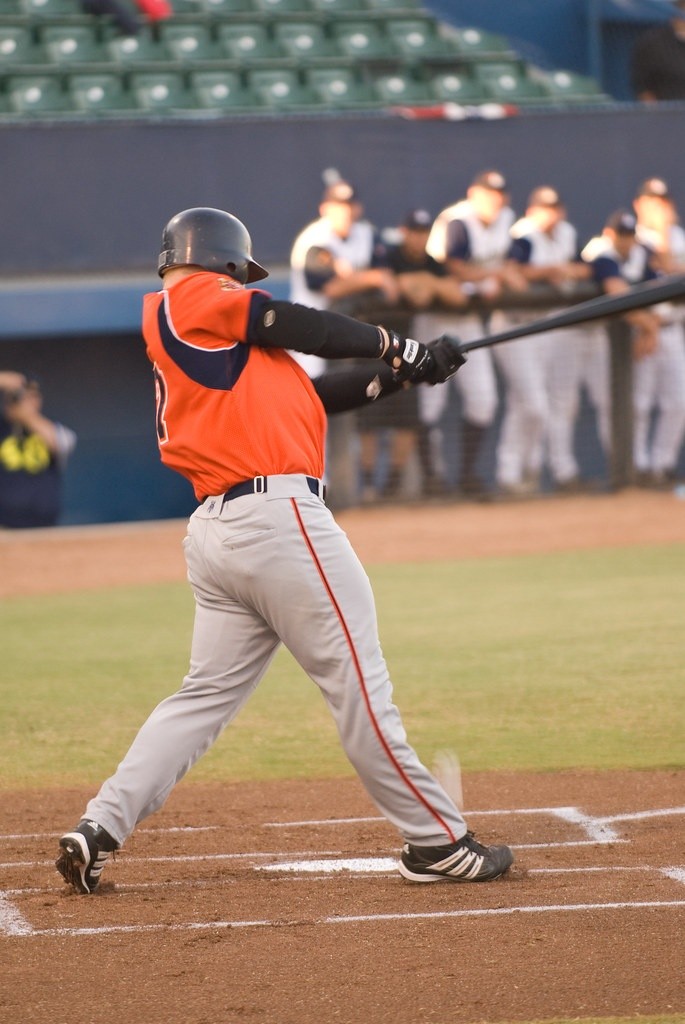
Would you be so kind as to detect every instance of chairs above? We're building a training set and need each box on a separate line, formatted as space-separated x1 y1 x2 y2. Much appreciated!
540 69 614 104
160 25 223 60
476 65 549 103
215 23 283 59
274 22 351 70
102 26 165 62
0 0 433 25
452 28 522 65
247 71 315 106
42 27 107 63
332 22 417 72
69 75 135 110
9 76 69 111
431 73 500 104
384 21 455 73
190 73 253 107
372 71 437 105
131 73 194 109
0 27 42 64
305 68 372 102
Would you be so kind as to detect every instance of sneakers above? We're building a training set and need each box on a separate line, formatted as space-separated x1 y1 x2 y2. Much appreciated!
395 830 513 883
55 819 121 895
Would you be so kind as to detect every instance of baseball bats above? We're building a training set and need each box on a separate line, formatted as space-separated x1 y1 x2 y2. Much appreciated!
407 270 685 384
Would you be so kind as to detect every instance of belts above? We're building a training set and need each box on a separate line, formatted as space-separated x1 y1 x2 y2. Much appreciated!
201 476 327 503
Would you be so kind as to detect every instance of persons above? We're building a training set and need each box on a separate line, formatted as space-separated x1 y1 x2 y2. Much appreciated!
629 0 685 103
78 0 142 39
281 175 685 509
54 207 514 898
0 371 80 528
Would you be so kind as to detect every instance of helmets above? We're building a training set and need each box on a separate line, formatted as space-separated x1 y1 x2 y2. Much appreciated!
156 207 269 285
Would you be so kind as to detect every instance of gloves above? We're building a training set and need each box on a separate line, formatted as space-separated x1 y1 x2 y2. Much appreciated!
374 324 466 386
